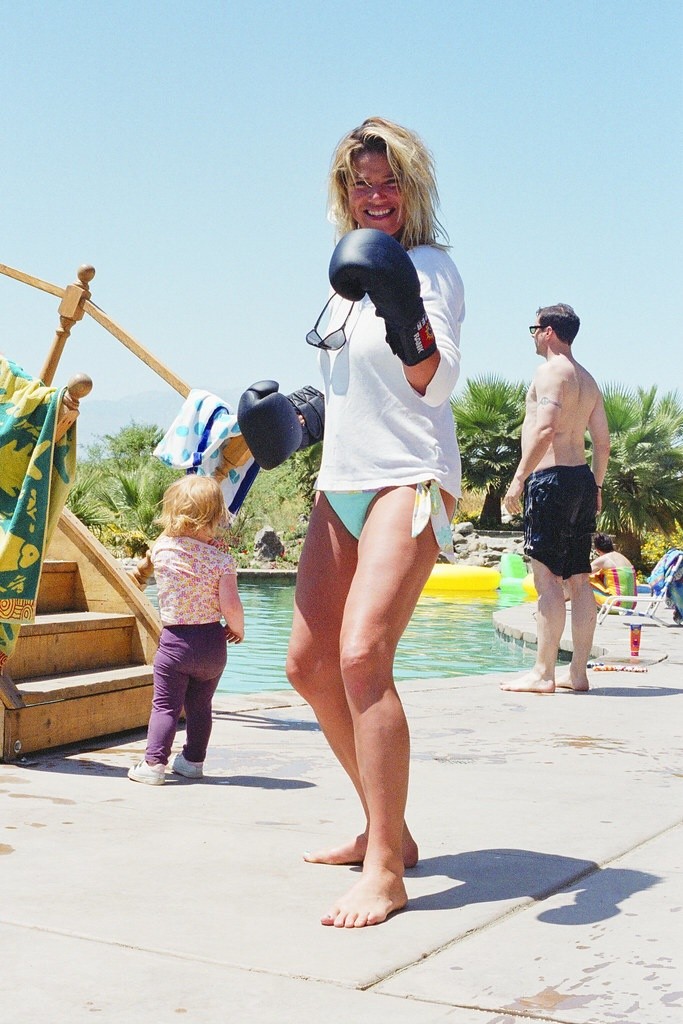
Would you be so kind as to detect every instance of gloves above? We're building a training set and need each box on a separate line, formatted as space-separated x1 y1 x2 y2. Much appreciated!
328 229 437 366
237 380 325 470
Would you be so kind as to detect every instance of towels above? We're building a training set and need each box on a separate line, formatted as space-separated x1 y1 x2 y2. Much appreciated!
2 353 78 680
646 549 683 624
151 388 262 516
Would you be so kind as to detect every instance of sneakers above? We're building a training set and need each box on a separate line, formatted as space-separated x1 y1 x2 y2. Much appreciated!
166 751 204 779
127 759 166 784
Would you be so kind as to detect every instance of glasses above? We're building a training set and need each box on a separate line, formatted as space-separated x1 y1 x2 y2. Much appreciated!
306 292 355 350
530 326 548 334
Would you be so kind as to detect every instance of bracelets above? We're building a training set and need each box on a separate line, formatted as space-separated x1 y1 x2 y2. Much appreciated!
597 485 602 489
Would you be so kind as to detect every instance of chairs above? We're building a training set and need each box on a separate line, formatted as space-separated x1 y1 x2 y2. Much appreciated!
596 547 683 629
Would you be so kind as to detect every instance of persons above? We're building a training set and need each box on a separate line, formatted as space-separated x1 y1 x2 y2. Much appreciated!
238 116 466 929
128 475 245 786
501 305 609 693
536 533 637 615
